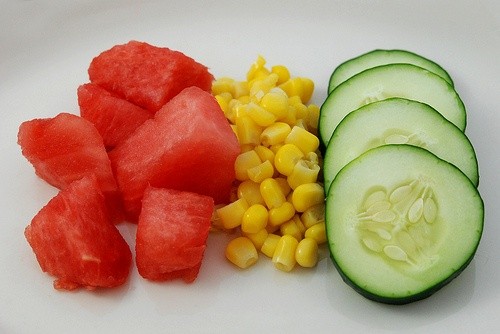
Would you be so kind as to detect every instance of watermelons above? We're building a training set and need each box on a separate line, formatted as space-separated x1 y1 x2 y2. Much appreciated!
18 41 240 290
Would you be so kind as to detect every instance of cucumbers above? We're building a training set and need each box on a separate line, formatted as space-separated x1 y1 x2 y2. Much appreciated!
317 49 486 306
